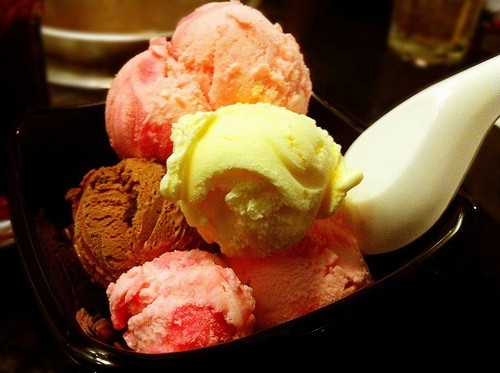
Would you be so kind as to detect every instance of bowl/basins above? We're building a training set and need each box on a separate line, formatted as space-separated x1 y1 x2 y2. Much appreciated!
11 85 465 372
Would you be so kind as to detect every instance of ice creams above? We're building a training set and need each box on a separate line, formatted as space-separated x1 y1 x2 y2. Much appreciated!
63 0 372 355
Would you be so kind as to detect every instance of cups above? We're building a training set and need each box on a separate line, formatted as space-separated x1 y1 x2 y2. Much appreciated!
388 1 483 66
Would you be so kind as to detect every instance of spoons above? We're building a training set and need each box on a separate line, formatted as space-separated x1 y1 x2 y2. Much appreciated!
341 54 500 256
